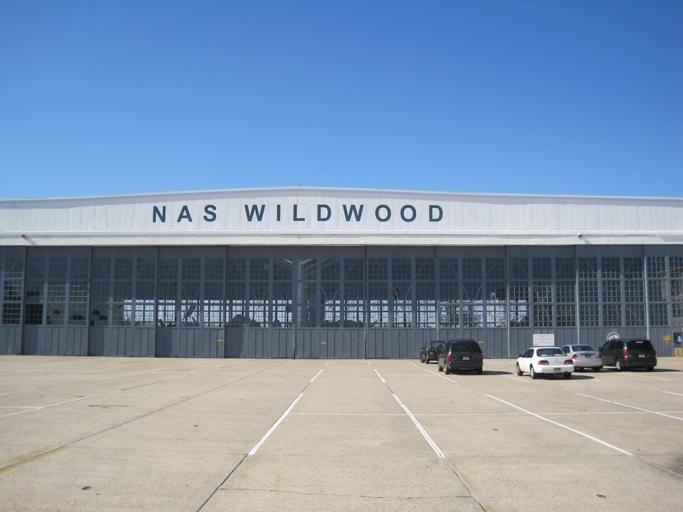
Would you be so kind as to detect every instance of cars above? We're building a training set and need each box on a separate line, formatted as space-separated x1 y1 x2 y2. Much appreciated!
515 346 574 380
562 344 602 374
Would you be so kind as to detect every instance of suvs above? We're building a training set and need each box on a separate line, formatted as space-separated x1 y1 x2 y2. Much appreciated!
419 339 445 364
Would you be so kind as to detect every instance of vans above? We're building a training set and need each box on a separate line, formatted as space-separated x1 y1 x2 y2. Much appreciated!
598 337 656 372
438 339 483 374
